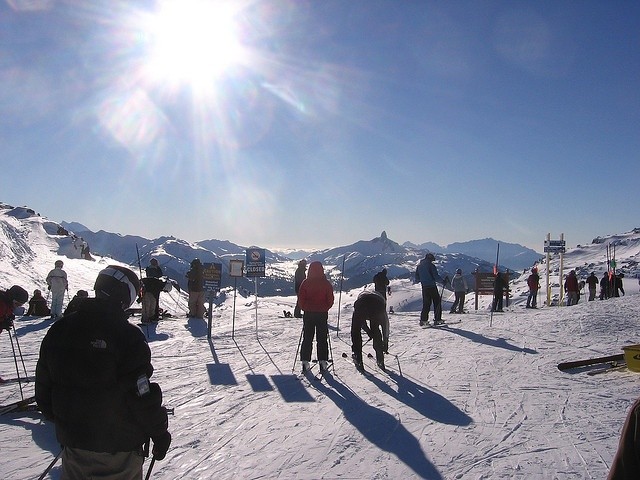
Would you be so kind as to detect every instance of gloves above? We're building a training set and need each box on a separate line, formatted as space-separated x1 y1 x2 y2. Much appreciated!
48 286 51 289
149 417 171 461
7 311 15 320
2 318 13 331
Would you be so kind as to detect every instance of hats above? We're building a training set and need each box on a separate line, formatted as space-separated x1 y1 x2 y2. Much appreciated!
5 285 29 303
149 258 158 265
382 269 388 275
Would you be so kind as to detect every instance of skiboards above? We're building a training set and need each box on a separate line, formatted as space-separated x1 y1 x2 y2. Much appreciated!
0 396 41 416
557 353 624 375
342 352 399 384
296 360 333 381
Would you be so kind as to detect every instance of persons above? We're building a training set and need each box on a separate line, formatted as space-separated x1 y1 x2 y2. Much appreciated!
0 285 28 334
186 259 205 318
294 260 307 318
577 281 585 304
586 272 598 301
564 270 578 306
612 273 624 297
146 259 162 278
35 265 172 480
416 253 444 326
298 261 334 371
351 291 389 363
373 269 389 299
46 260 68 320
600 272 609 300
65 290 88 315
526 268 539 309
491 271 509 312
23 290 51 316
450 269 468 314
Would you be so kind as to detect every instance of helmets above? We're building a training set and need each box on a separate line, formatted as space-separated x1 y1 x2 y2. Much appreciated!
55 261 63 268
456 268 462 273
192 257 199 267
94 265 139 309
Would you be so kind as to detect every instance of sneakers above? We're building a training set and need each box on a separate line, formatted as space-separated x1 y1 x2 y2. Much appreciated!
352 354 362 364
320 360 328 371
491 309 496 311
526 305 531 308
301 361 310 371
497 309 503 312
459 310 465 313
449 311 456 313
376 351 384 363
419 320 429 326
434 319 445 325
532 306 536 308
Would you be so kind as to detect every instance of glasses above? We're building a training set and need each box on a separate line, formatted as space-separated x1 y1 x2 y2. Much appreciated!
9 295 24 306
